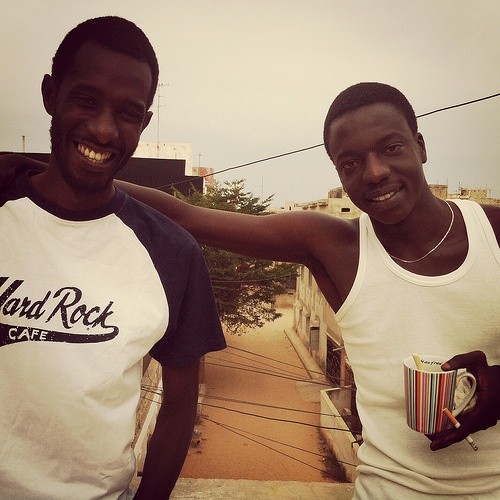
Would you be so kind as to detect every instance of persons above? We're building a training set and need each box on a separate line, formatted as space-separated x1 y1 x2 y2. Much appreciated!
0 16 226 500
0 83 500 500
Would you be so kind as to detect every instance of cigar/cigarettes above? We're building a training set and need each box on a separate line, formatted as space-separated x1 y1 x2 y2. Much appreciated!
442 408 478 451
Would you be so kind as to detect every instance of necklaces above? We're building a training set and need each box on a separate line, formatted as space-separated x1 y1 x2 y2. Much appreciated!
388 200 454 262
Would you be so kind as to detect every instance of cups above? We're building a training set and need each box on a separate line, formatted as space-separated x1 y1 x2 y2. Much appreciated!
403 355 477 434
